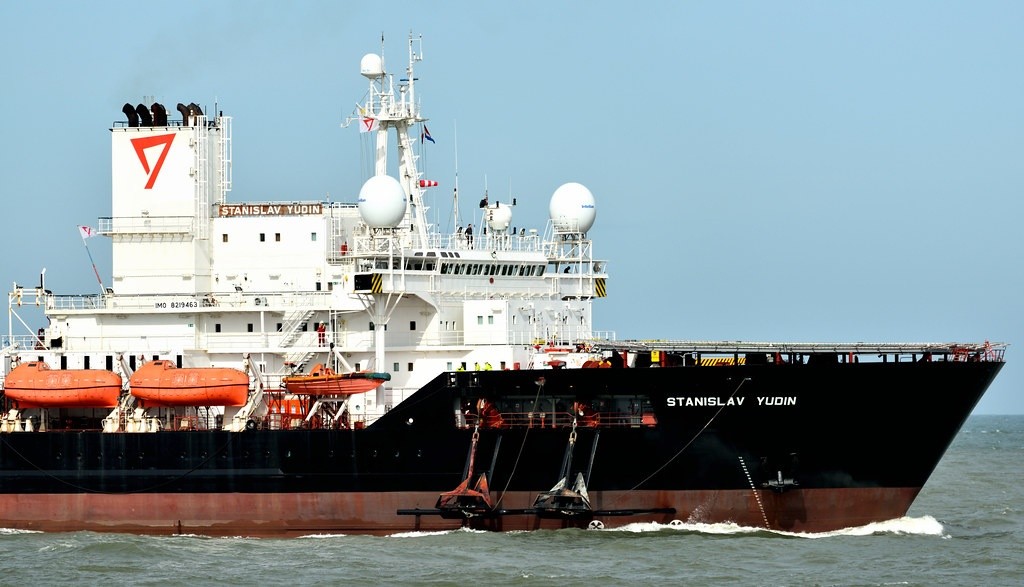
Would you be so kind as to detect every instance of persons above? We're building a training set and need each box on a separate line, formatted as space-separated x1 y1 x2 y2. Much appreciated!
483 362 493 371
593 262 601 272
564 266 571 273
457 366 465 372
474 362 480 371
464 224 474 251
317 320 326 347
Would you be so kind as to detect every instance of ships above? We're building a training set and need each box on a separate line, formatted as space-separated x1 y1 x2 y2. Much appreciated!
0 29 1012 536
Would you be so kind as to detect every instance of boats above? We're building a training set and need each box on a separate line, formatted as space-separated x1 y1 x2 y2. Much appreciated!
130 360 249 410
4 361 119 411
280 369 392 398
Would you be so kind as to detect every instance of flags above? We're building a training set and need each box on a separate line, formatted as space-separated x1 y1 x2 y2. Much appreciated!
77 225 97 239
356 108 381 134
421 124 436 145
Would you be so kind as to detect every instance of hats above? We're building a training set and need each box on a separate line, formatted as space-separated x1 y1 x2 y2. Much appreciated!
320 321 324 323
